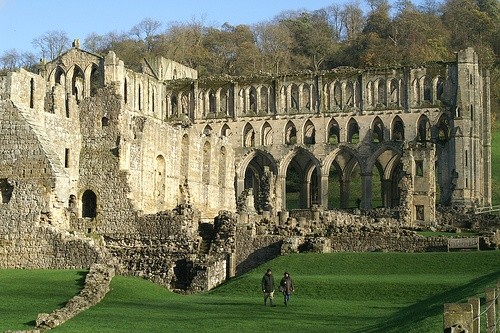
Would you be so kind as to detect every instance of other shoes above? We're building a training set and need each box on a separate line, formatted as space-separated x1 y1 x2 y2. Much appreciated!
283 302 288 308
265 302 267 306
270 304 277 307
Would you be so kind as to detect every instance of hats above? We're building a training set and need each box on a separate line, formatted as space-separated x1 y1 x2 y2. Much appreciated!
284 272 289 275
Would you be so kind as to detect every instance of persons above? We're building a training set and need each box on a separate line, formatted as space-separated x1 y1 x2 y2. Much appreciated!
280 271 295 307
261 269 279 306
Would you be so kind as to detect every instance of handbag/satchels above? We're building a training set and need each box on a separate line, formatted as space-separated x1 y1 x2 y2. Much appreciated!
278 280 287 292
